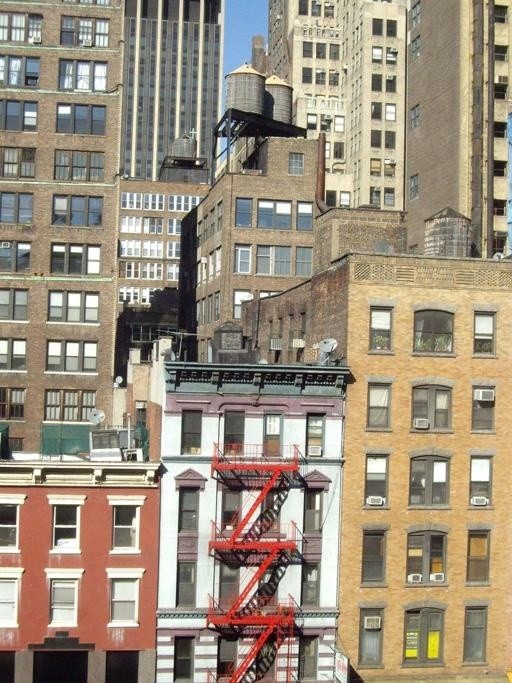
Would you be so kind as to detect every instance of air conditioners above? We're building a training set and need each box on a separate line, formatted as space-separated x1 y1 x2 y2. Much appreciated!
83 39 92 47
408 574 423 583
413 418 428 429
364 616 382 629
390 159 396 164
32 36 41 44
308 445 322 456
325 114 332 119
366 496 386 505
387 74 393 80
474 390 494 401
391 47 398 52
430 573 444 582
470 497 489 505
